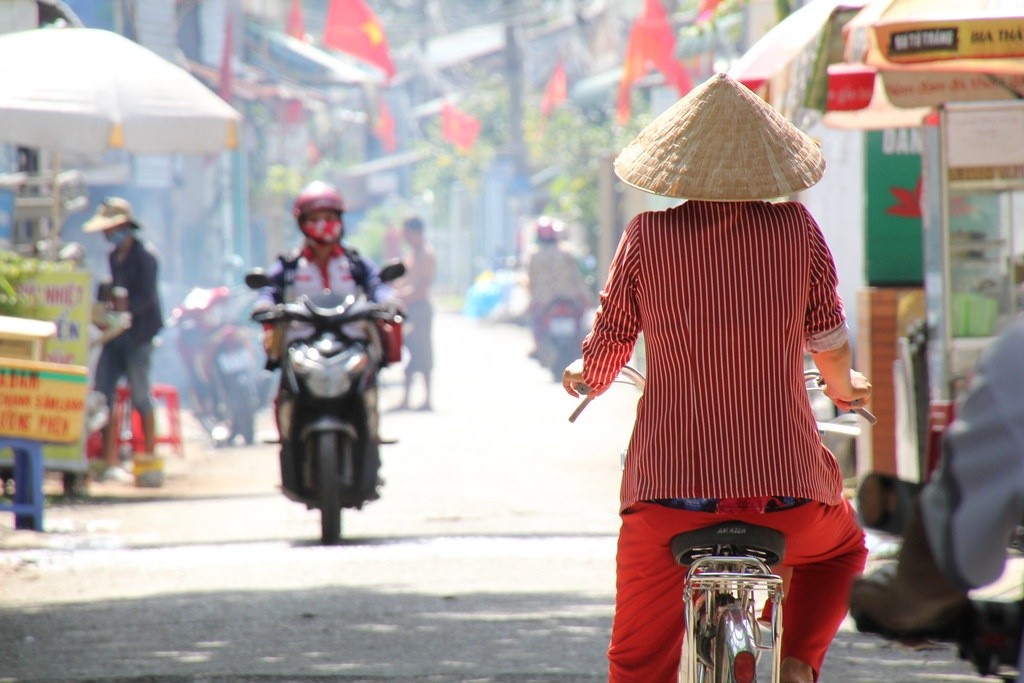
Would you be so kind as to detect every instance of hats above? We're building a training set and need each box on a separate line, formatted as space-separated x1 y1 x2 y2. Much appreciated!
613 72 826 202
81 197 139 233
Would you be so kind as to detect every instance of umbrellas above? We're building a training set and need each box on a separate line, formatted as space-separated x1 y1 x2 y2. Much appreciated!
0 18 244 152
730 0 1024 383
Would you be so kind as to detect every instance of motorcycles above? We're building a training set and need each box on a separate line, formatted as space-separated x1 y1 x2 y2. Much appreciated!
152 257 272 447
506 255 597 383
245 254 406 542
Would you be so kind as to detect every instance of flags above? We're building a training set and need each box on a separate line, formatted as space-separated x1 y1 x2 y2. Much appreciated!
616 1 694 122
202 0 395 169
442 104 480 151
539 62 568 122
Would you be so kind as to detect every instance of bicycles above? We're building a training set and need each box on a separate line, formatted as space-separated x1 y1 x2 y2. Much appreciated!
569 365 881 683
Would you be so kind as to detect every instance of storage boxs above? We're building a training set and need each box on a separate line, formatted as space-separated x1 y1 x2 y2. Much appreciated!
0 355 89 444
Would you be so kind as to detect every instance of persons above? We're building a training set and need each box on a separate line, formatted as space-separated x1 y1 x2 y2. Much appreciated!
848 315 1024 636
396 217 436 410
251 180 393 446
82 197 164 482
563 71 872 682
529 218 586 359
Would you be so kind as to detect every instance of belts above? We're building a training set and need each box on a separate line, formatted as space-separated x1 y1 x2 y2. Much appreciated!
643 496 810 513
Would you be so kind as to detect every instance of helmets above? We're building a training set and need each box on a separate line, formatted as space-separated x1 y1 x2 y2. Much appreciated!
537 219 554 238
294 181 345 221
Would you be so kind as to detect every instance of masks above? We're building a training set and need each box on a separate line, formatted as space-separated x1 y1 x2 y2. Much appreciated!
300 223 342 244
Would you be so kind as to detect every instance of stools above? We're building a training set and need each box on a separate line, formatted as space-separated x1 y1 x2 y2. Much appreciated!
0 438 45 532
114 385 183 461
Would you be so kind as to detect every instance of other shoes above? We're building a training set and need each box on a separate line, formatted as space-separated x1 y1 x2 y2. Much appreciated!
134 469 163 487
